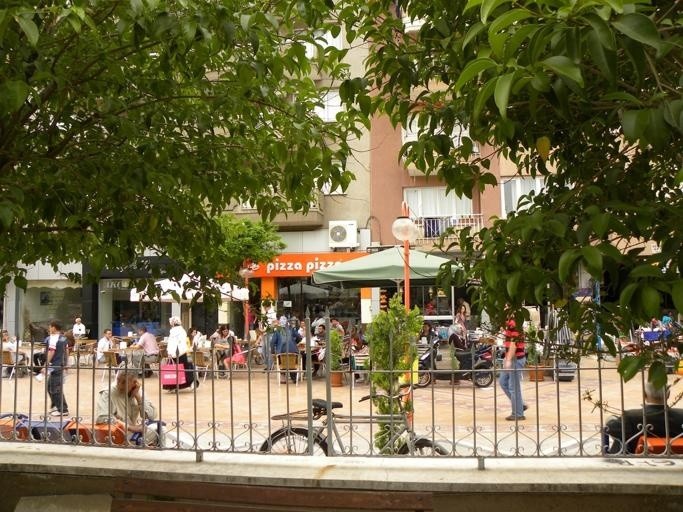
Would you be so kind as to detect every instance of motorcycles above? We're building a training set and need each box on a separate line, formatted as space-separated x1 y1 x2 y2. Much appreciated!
409 335 494 388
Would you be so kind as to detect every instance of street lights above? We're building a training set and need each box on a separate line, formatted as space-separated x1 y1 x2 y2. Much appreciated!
392 200 420 428
239 252 261 338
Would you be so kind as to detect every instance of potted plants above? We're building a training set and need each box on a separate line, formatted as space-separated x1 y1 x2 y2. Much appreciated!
525 322 545 382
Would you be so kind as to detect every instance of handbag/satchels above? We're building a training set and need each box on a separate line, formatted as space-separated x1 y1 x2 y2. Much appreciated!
161 364 187 385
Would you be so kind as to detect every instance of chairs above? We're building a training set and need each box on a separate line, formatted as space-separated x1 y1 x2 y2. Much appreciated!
629 319 683 353
611 428 683 455
449 329 524 380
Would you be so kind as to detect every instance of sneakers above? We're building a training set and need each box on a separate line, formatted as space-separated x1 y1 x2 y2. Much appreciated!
47 406 69 415
190 380 198 390
168 389 177 393
505 404 527 420
138 371 153 377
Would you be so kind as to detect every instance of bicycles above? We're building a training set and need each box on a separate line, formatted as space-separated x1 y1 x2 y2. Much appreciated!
258 384 451 459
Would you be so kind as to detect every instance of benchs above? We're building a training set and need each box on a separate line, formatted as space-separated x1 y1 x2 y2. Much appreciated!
0 410 166 449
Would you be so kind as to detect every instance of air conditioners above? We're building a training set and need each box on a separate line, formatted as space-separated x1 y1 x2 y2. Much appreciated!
329 221 358 249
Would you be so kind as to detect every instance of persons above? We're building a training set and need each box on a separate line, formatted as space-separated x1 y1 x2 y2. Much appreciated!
421 297 470 385
96 329 121 374
41 320 69 415
499 303 528 420
3 318 85 375
256 316 345 383
605 381 683 454
187 324 244 379
97 372 166 447
651 313 674 332
163 317 199 393
130 326 158 377
